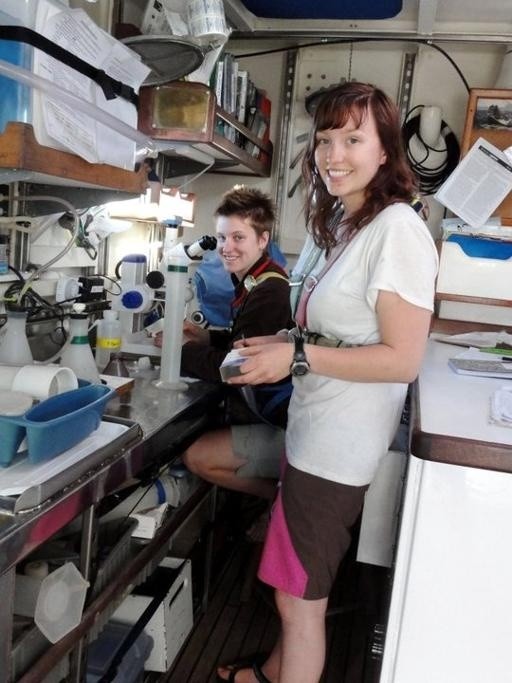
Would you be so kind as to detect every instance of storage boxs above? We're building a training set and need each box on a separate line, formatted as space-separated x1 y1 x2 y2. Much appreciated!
109 556 196 673
85 619 155 681
435 236 512 306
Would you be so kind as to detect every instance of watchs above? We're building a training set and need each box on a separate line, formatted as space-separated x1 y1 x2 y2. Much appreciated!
290 336 311 376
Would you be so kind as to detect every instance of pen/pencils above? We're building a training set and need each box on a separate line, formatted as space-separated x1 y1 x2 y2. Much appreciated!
502 356 512 360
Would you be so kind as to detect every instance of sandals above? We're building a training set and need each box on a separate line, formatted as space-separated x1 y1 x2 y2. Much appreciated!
214 662 273 683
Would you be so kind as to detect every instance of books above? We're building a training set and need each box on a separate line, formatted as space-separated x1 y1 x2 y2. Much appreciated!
447 358 512 379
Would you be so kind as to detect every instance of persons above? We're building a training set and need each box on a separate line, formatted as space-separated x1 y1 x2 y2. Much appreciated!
153 185 295 544
216 78 440 683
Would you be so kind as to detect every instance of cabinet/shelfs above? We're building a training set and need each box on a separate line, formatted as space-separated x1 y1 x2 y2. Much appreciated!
0 362 231 682
